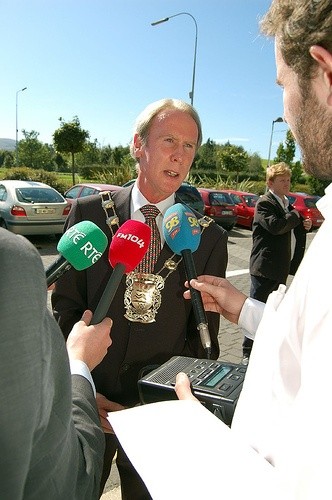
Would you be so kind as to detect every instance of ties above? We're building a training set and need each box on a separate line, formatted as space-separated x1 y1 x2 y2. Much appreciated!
132 204 161 274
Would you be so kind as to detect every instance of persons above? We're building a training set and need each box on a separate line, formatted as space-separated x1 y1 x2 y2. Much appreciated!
47 98 230 500
0 228 114 500
173 1 332 499
240 162 313 366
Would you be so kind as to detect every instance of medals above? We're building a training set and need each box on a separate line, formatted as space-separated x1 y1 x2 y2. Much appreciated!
124 272 165 324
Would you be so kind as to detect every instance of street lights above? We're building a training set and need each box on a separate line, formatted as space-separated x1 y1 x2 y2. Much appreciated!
266 116 283 168
151 11 199 106
16 86 28 147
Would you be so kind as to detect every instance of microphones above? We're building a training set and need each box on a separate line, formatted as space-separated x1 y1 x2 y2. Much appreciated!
89 219 151 325
45 220 108 288
163 203 212 354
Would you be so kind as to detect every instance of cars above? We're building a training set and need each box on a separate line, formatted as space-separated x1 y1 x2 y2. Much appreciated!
223 189 261 231
61 183 123 208
287 191 325 233
196 187 238 231
0 179 71 243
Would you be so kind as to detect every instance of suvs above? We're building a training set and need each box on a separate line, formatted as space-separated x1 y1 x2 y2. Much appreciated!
122 178 204 215
